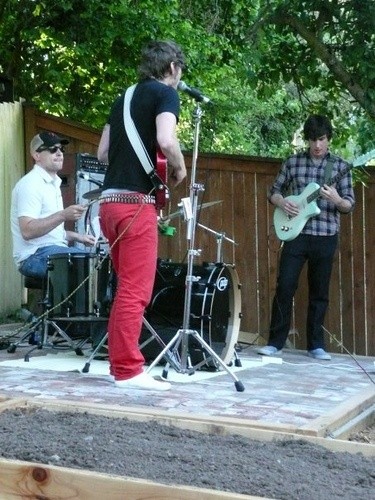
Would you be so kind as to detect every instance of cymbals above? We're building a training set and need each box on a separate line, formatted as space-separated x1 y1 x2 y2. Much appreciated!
157 200 225 222
83 188 102 200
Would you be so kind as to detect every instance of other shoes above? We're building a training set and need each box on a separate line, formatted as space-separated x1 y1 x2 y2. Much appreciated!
307 348 331 360
257 345 282 355
115 372 171 390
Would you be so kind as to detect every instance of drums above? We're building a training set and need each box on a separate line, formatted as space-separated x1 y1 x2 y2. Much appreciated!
139 258 242 372
45 252 118 321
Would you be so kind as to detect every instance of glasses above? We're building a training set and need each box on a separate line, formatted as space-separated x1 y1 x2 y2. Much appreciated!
40 144 66 154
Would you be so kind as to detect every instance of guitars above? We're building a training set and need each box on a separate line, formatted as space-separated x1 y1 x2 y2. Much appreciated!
156 148 169 210
273 148 375 241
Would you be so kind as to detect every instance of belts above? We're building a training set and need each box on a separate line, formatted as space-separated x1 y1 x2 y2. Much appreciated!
99 193 155 206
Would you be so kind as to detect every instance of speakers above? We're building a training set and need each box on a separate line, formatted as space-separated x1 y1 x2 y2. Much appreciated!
55 153 112 254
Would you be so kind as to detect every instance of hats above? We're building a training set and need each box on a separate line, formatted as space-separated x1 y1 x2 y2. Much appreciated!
30 132 70 154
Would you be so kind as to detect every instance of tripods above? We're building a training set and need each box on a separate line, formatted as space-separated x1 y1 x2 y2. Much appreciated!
81 103 246 393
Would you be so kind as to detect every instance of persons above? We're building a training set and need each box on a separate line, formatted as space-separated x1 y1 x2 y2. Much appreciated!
97 40 187 390
256 113 354 360
10 132 98 278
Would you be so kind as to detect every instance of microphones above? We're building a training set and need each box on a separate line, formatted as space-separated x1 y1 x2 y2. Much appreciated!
177 81 214 105
78 173 104 187
